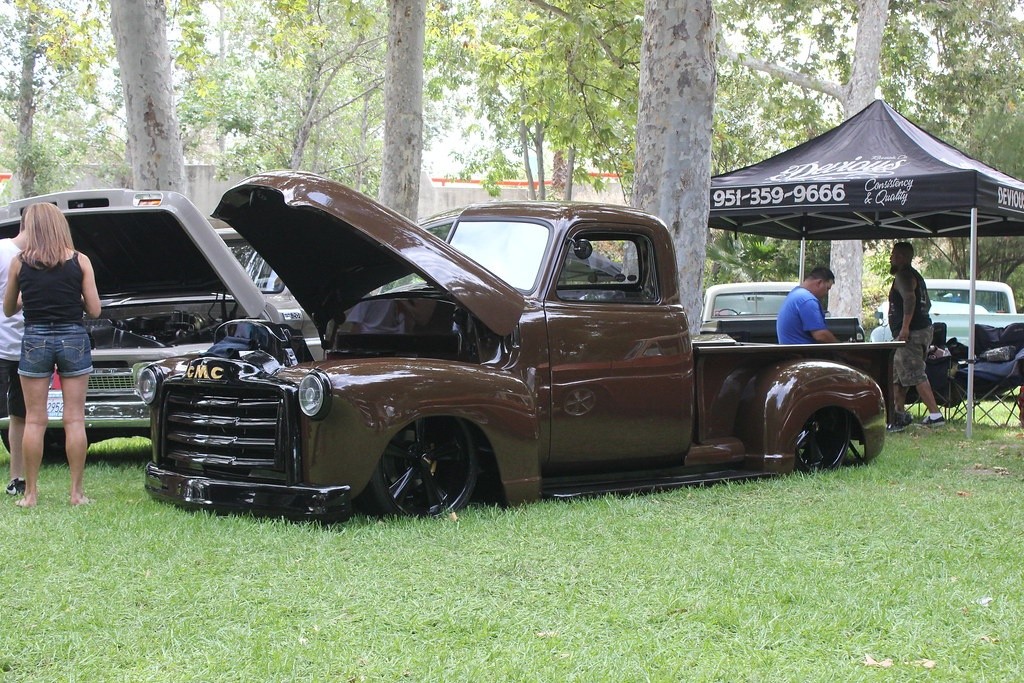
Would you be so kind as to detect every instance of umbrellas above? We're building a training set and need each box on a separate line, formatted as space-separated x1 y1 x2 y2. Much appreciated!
559 244 621 282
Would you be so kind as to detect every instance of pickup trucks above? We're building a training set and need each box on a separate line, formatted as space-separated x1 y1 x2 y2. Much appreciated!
142 162 890 529
698 281 867 344
871 277 1024 356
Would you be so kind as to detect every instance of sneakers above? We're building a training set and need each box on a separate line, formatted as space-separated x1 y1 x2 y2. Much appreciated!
885 411 912 433
919 416 945 428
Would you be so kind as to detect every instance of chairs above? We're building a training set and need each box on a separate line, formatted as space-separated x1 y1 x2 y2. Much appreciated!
906 322 1024 429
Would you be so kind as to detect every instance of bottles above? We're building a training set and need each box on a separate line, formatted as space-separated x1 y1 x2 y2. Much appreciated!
943 348 952 357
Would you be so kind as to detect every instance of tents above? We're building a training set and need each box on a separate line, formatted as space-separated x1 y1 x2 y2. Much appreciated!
708 99 1024 438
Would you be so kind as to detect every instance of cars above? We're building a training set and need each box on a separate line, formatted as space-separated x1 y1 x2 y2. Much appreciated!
0 186 328 460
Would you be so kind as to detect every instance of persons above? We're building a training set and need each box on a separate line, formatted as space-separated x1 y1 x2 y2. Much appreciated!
776 266 839 345
887 242 946 433
4 202 101 508
0 206 27 495
347 288 454 336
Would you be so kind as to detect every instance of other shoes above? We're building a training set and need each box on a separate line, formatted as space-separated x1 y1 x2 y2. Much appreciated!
5 477 25 495
